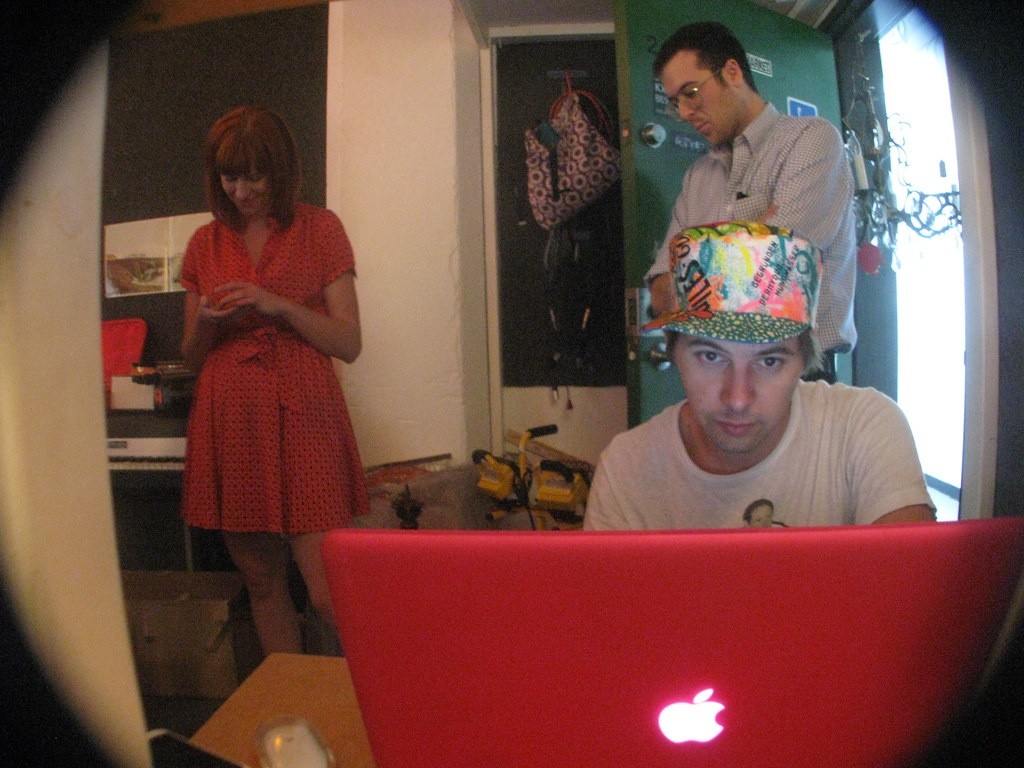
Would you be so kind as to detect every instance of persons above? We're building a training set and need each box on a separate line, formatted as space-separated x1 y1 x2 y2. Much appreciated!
583 220 937 532
648 20 858 385
179 106 371 656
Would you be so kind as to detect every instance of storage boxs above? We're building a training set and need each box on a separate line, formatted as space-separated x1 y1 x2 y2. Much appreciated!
123 567 264 700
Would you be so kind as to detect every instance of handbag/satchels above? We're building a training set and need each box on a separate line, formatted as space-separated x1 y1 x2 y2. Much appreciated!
523 68 621 232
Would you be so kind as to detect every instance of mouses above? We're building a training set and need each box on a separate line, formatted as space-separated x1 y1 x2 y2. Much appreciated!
256 720 327 768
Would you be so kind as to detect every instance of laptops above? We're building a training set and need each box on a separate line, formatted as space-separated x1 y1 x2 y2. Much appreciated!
321 519 1024 768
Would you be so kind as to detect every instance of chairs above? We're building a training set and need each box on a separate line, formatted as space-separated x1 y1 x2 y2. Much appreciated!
101 316 149 392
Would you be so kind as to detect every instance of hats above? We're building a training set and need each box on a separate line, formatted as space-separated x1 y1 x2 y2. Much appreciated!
642 220 825 344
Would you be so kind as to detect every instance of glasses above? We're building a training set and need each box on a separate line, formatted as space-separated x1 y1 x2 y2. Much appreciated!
665 70 721 122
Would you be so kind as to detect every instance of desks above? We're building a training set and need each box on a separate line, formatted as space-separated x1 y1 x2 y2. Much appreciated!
190 652 375 768
112 460 206 571
